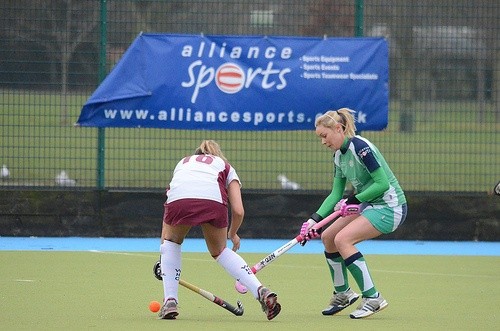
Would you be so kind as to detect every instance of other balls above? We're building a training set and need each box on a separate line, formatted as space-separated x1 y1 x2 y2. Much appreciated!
149 301 160 312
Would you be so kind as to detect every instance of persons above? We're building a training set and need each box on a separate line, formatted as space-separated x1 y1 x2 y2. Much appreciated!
299 109 407 320
158 140 282 320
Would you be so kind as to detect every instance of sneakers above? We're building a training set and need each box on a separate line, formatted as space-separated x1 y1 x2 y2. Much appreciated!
322 287 360 315
350 292 389 319
258 286 281 320
158 298 179 320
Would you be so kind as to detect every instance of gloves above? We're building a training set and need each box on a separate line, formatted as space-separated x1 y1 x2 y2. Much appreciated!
333 195 360 217
300 218 322 246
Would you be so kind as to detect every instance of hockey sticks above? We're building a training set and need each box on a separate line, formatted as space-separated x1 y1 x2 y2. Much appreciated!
235 210 341 294
157 268 244 317
